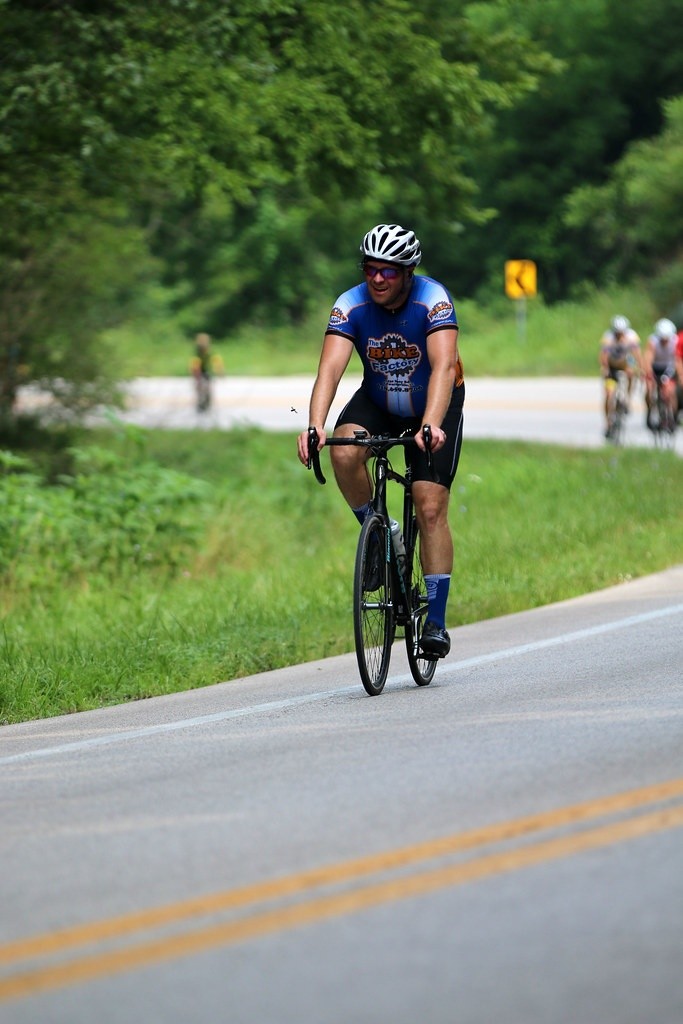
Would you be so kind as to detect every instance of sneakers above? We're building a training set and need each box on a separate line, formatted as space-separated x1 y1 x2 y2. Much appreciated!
364 548 385 591
420 623 451 656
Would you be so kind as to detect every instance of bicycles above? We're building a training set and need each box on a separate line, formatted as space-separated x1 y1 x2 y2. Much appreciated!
645 368 677 446
307 423 444 696
605 369 636 443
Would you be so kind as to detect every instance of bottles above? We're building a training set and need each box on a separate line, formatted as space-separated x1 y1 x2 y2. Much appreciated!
388 515 406 561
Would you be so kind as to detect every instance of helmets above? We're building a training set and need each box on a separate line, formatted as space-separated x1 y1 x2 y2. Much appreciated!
654 318 676 341
611 315 628 333
360 223 423 266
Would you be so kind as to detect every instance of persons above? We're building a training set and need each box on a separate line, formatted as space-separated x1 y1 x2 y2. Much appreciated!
298 225 465 656
600 314 644 437
193 333 222 395
644 317 683 432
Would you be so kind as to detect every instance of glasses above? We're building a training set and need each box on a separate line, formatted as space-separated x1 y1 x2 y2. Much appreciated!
362 263 400 279
